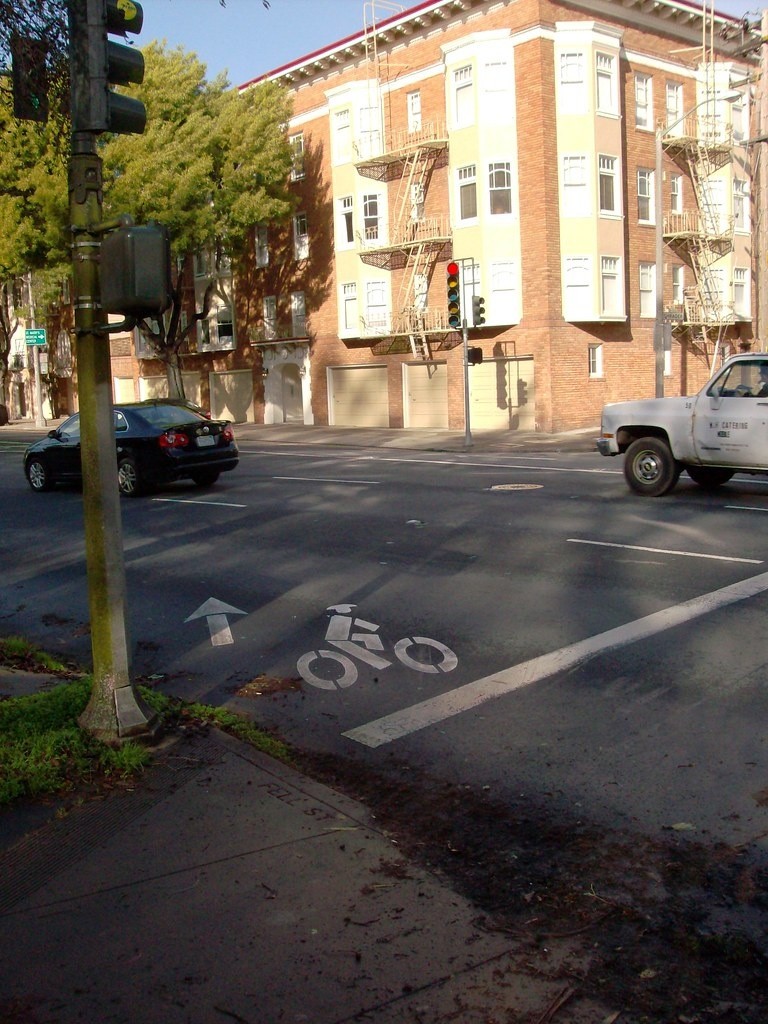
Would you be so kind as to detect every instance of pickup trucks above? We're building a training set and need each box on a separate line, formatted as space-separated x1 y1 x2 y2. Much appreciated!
595 352 768 498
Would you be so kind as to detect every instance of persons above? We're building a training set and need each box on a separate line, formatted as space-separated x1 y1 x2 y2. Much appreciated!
738 361 768 397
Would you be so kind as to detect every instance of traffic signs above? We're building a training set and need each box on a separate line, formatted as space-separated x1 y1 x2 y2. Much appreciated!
24 329 47 345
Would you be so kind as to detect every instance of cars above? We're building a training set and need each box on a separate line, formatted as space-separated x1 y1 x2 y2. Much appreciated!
142 397 211 422
23 401 240 498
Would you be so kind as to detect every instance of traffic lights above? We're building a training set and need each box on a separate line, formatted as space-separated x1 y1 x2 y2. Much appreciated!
100 0 147 133
472 295 485 325
468 347 483 363
446 261 461 328
